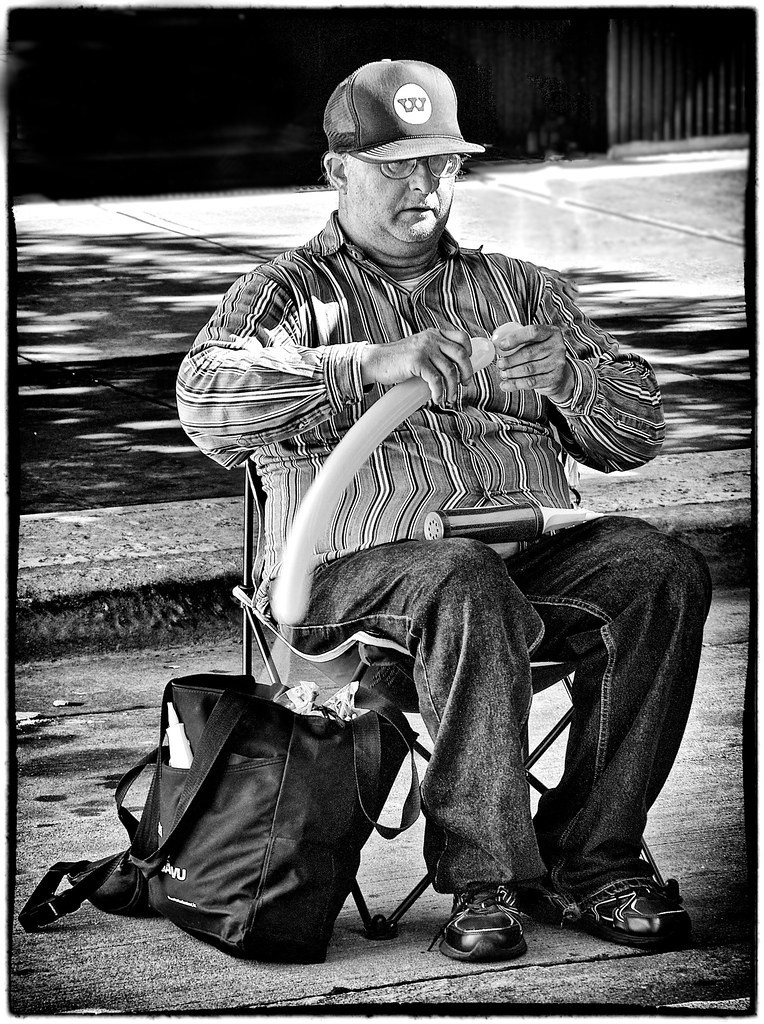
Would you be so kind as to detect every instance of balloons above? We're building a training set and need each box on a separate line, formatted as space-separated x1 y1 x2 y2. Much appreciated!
274 322 526 627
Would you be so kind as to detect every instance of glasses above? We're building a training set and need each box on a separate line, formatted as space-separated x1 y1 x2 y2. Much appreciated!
380 154 463 181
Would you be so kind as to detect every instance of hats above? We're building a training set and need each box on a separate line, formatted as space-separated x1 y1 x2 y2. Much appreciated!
324 59 486 164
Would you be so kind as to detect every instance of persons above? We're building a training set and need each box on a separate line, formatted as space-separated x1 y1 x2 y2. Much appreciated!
176 58 713 959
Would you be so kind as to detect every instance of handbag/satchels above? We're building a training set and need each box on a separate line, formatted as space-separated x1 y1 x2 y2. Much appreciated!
16 674 418 961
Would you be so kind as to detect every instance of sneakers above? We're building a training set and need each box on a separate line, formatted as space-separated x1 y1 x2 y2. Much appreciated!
429 871 526 961
516 859 691 949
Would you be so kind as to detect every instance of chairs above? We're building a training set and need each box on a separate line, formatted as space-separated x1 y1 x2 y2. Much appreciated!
230 451 686 942
